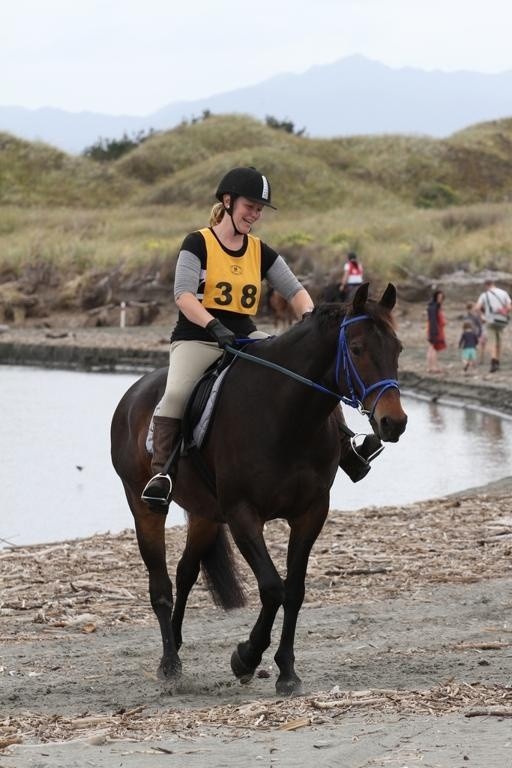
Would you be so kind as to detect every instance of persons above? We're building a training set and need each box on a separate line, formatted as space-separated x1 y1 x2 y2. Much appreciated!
425 290 448 373
338 252 365 293
474 278 512 373
457 323 478 372
138 164 384 508
459 300 490 363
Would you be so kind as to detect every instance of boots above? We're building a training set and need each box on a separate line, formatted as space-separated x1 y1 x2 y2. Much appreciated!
143 415 184 515
331 400 384 483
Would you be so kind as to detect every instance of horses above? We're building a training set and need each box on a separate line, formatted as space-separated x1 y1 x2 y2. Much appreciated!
103 278 408 701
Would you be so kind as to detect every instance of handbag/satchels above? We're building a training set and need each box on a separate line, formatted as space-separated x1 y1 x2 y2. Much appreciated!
487 313 509 327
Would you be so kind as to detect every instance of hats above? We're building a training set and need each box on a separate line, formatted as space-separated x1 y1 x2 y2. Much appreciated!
215 166 279 212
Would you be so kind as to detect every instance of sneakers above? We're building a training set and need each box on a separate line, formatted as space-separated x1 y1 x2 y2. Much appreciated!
462 358 501 374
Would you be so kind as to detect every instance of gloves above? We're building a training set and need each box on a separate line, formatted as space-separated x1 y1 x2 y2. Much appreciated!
203 318 239 350
